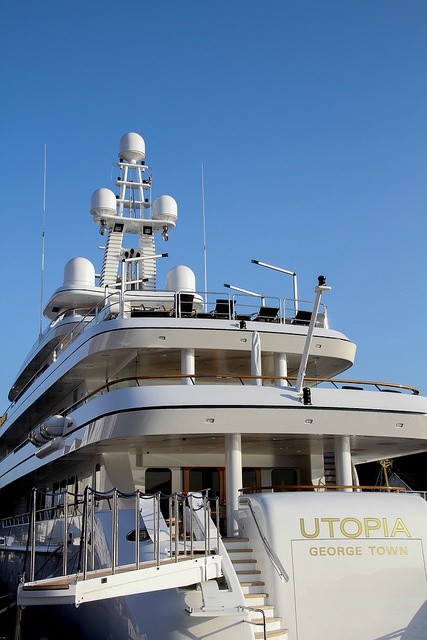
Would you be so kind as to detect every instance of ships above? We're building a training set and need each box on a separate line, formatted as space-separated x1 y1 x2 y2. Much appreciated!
0 133 427 640
